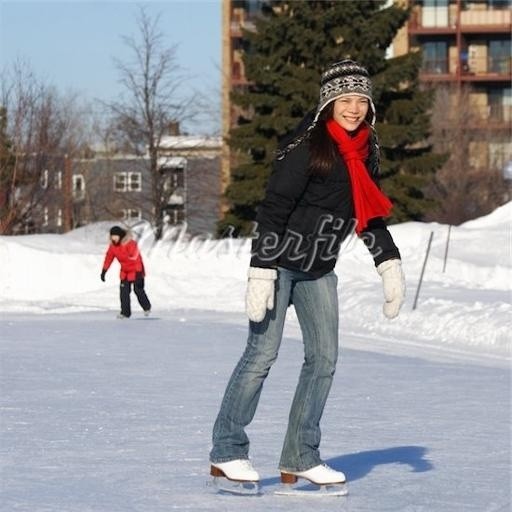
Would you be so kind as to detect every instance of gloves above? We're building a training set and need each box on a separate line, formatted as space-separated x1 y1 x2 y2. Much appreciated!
135 271 144 289
244 266 277 323
101 269 106 282
376 257 406 320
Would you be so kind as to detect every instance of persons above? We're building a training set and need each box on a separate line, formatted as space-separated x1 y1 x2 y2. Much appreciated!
209 54 407 485
100 225 151 318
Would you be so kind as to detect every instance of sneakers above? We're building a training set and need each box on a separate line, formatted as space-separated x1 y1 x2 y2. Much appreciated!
117 314 125 319
144 309 150 317
209 459 260 483
280 462 347 485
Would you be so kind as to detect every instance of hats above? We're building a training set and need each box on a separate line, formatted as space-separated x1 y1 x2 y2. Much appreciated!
312 58 377 132
110 226 123 240
